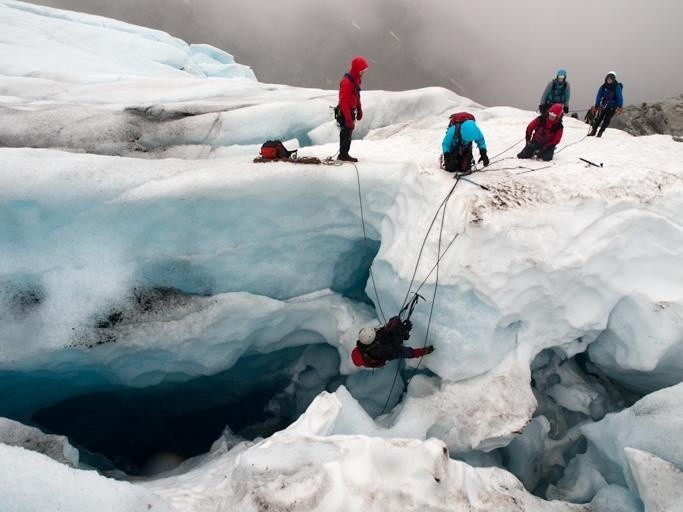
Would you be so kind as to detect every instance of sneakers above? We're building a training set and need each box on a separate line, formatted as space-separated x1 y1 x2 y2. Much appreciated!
338 155 357 162
426 345 434 354
587 129 602 137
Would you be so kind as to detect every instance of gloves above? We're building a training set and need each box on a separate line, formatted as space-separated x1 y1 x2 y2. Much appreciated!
347 120 354 129
563 106 568 113
357 111 362 120
539 105 545 113
615 107 623 114
478 149 489 167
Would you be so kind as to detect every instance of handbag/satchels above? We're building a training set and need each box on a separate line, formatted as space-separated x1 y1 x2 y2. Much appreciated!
261 140 290 159
351 348 385 369
448 112 475 125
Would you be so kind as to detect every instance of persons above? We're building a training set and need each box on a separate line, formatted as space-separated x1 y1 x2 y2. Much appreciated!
441 119 489 172
352 317 434 368
539 69 571 113
586 71 624 137
517 103 564 161
336 56 369 162
584 106 596 126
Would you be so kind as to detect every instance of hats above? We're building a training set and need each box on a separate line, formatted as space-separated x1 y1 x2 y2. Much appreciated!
359 328 376 345
558 76 564 78
549 112 556 116
